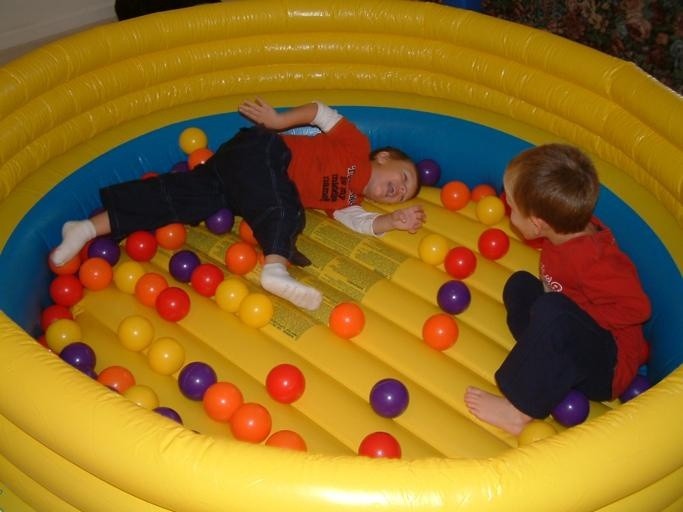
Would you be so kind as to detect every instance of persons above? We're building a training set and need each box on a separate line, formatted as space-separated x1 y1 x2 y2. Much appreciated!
50 99 429 312
463 142 653 438
